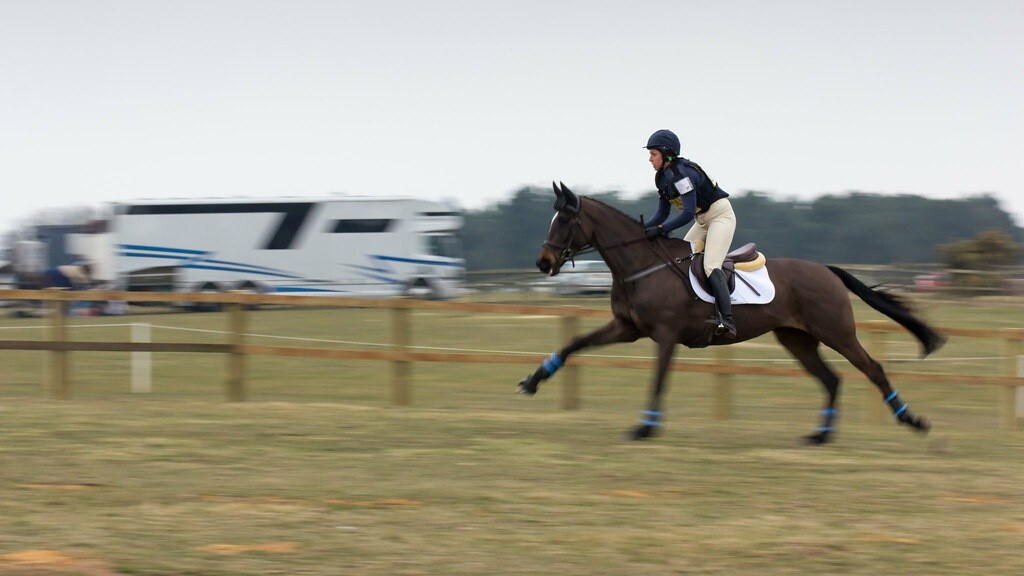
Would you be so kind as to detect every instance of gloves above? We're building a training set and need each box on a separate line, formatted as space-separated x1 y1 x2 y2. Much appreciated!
644 226 663 241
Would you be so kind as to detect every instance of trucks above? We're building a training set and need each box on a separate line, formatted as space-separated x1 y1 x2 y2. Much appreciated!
110 197 464 313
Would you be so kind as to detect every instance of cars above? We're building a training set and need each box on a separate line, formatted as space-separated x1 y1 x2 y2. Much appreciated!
547 260 613 296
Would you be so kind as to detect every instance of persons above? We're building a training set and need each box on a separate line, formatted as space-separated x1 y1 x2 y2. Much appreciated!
642 130 736 337
46 265 91 287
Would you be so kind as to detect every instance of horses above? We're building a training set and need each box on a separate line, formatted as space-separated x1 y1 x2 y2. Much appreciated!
513 180 949 446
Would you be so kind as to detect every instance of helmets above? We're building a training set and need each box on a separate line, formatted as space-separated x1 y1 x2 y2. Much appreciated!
642 129 680 157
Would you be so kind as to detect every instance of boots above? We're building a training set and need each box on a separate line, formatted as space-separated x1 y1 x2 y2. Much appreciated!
704 268 737 341
